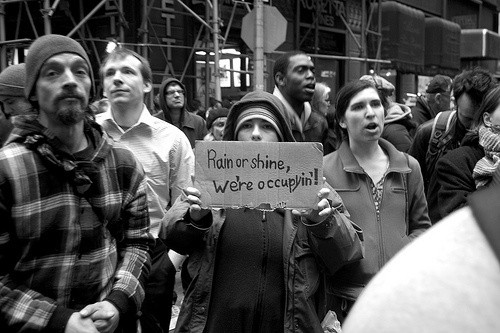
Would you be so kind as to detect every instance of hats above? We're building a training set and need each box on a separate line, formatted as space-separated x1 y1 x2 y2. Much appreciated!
425 74 453 93
0 63 27 95
234 102 285 142
359 73 395 96
206 108 229 130
24 34 95 100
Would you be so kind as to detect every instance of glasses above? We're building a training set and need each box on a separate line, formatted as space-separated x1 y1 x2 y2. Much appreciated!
167 90 185 94
325 99 333 103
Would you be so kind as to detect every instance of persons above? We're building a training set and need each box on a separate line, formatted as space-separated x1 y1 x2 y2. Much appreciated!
88 48 197 333
192 51 344 157
318 79 432 327
360 69 500 224
158 90 365 333
340 156 500 333
1 34 155 333
152 77 208 149
1 62 45 146
